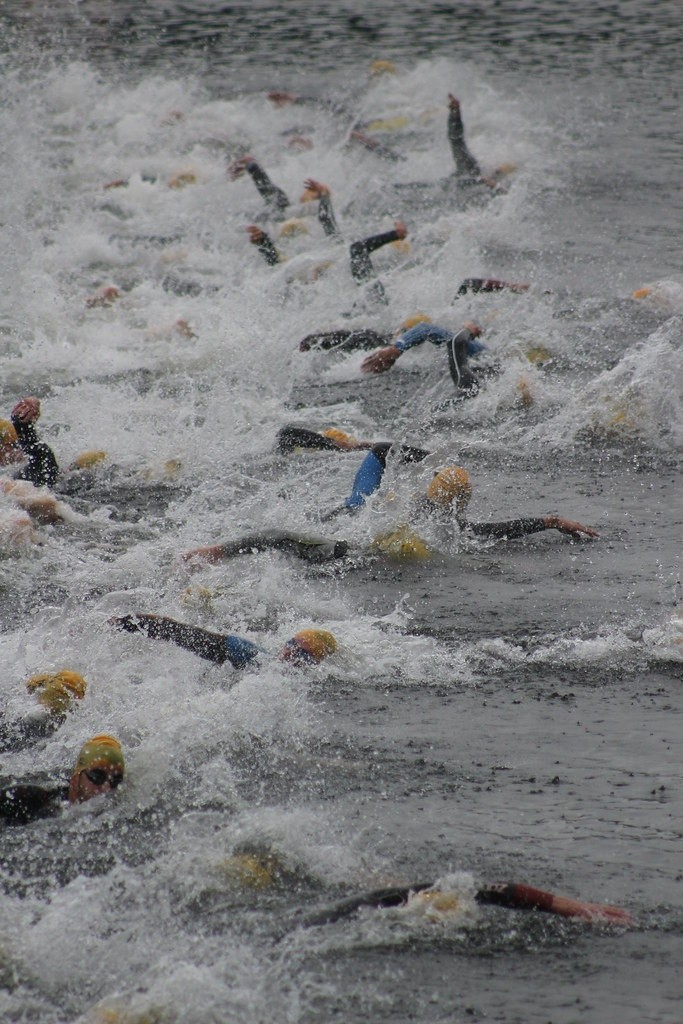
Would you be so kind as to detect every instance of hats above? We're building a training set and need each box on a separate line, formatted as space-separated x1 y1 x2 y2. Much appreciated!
73 736 125 773
27 669 86 710
403 314 429 328
381 534 429 561
530 347 551 368
301 186 319 201
372 61 395 71
324 428 357 448
69 453 104 469
278 218 307 238
425 467 472 512
296 630 336 660
170 174 195 189
0 419 20 443
635 288 649 298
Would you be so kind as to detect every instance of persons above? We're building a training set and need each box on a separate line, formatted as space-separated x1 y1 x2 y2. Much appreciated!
0 671 88 754
299 219 654 429
105 586 336 676
229 58 516 267
191 839 641 949
0 736 125 834
182 421 601 581
0 396 182 550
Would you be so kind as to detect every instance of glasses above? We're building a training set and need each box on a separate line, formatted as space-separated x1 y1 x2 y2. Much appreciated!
72 463 79 469
434 470 439 477
82 767 123 788
286 638 311 663
4 444 22 451
